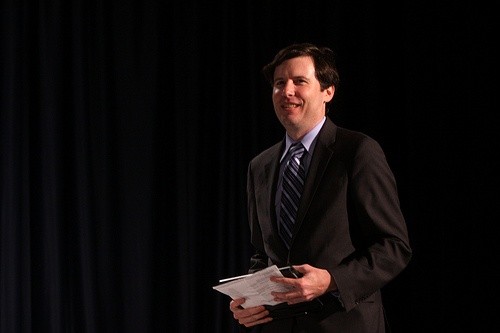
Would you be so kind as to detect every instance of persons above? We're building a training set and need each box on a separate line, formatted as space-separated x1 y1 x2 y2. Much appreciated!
228 46 411 332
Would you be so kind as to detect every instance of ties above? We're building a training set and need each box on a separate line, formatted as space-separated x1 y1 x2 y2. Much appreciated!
276 143 307 256
